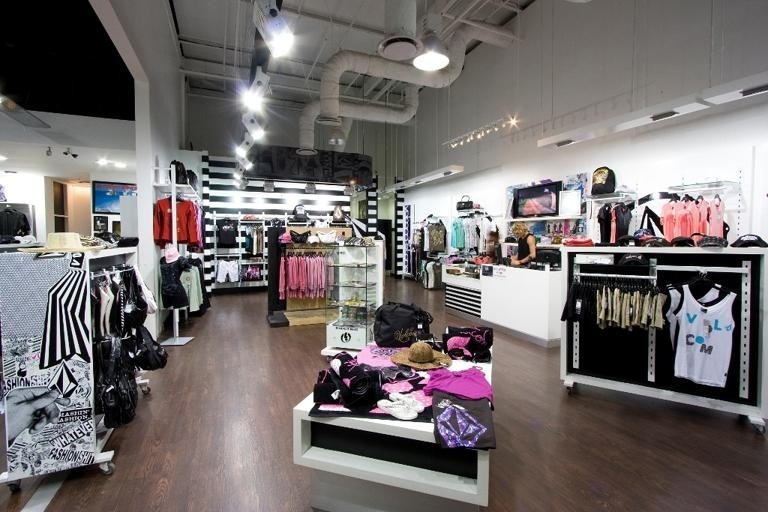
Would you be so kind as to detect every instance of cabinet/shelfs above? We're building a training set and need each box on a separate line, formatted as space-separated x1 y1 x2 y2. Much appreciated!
319 238 386 363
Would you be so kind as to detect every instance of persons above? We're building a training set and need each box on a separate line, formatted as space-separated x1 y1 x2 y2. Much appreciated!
158 247 192 309
5 386 71 448
510 221 537 268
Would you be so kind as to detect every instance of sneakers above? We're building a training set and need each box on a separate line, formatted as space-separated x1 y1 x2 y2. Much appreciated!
345 236 364 247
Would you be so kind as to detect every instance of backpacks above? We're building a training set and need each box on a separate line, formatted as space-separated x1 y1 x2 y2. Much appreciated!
591 166 615 194
333 205 345 221
187 169 199 192
169 160 187 184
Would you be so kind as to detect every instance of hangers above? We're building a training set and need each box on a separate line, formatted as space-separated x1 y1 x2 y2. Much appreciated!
157 190 196 206
598 199 630 213
280 245 333 261
664 269 738 297
661 190 727 212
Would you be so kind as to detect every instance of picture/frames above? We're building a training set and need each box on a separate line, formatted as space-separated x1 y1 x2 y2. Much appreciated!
91 180 137 216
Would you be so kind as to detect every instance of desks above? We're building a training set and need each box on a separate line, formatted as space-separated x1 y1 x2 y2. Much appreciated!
291 341 493 507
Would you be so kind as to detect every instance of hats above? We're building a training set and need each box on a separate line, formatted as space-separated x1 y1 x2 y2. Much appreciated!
633 228 670 247
730 234 768 248
16 230 104 253
670 235 695 246
390 340 452 370
697 236 728 247
96 232 140 245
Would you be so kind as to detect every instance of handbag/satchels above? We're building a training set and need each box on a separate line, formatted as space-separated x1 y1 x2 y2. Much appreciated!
455 194 473 211
374 300 434 348
124 265 157 329
135 321 169 369
93 337 138 429
317 229 337 243
290 229 310 244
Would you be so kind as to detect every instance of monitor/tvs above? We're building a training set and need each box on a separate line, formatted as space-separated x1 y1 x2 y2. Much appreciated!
535 246 561 270
512 181 563 217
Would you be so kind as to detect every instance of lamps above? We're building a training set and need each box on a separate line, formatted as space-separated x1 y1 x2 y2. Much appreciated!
450 120 506 148
304 182 316 194
344 185 354 196
46 147 78 159
411 1 451 72
231 0 296 193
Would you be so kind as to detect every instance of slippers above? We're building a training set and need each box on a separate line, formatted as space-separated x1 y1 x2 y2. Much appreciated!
376 392 426 421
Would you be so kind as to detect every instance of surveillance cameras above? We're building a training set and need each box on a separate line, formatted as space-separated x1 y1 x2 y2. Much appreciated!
46 146 52 156
